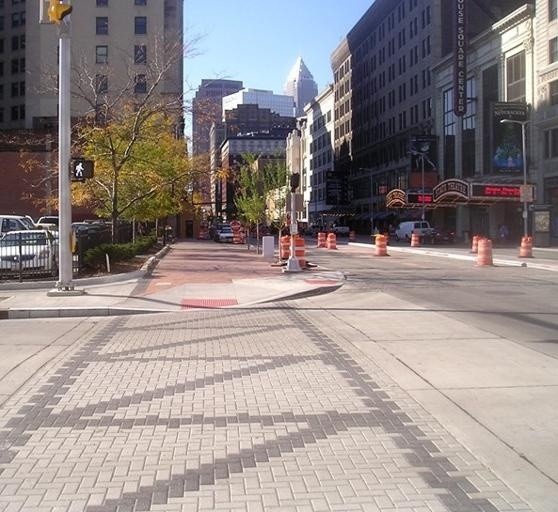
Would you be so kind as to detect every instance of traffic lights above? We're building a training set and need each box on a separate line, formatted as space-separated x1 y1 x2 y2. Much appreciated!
73 160 95 180
46 0 73 24
290 173 300 193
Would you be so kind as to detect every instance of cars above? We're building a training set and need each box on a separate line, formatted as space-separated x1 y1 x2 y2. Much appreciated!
0 214 131 280
307 223 350 236
208 224 233 243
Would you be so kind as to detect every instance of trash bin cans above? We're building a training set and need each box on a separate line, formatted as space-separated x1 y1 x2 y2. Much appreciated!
262 236 274 257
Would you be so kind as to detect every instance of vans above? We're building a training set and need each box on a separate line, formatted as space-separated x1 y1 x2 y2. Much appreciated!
394 220 431 242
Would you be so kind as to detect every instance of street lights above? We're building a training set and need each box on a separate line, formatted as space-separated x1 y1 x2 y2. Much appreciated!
359 168 376 235
406 150 427 221
500 118 534 238
